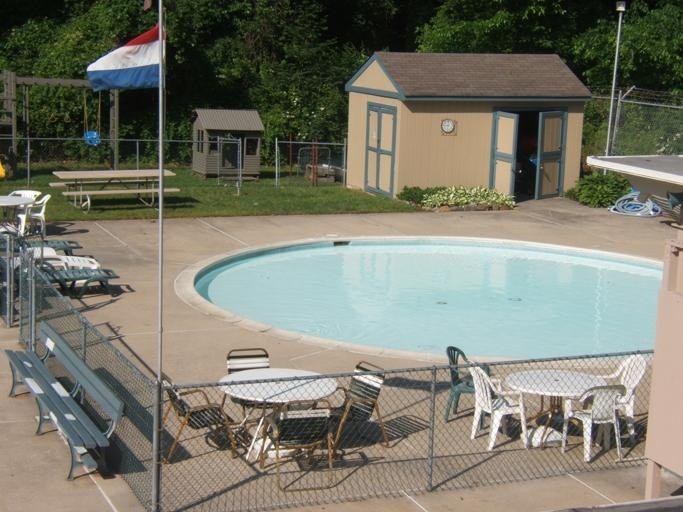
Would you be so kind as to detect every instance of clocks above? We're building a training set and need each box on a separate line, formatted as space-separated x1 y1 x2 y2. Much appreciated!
441 119 456 134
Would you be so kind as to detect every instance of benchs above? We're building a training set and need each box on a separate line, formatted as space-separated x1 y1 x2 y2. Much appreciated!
5 321 124 480
49 178 181 213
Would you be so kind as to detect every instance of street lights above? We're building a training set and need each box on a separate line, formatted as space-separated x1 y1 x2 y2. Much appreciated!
601 0 627 178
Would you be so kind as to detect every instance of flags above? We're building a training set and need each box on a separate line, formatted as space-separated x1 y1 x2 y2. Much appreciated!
87 23 163 92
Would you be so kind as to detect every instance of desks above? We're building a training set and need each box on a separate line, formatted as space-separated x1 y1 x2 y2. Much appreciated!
52 168 176 214
217 367 337 462
0 194 34 232
504 369 608 450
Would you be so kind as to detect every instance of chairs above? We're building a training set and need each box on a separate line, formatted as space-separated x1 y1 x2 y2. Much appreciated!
314 360 390 449
7 189 52 238
443 346 532 451
221 347 290 427
158 371 238 463
260 399 334 492
0 233 120 299
561 352 648 463
